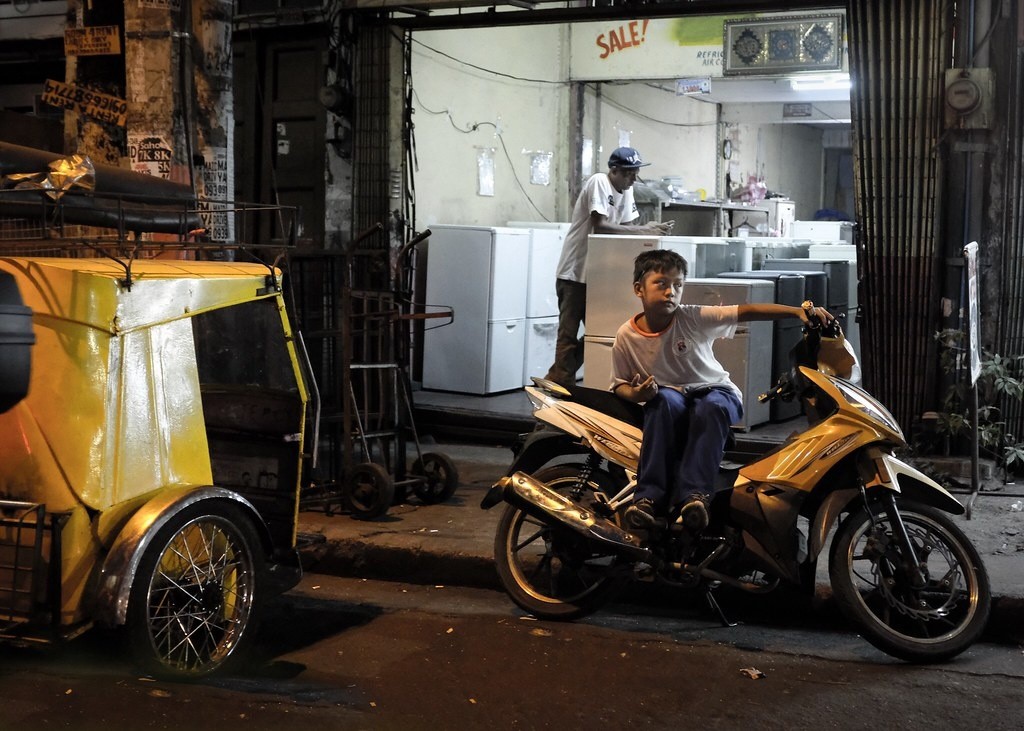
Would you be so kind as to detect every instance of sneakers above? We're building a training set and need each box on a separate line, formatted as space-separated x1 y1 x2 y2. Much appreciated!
624 500 668 532
676 493 709 531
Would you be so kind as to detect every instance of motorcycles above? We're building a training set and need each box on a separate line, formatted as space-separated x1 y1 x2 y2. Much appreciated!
480 299 994 666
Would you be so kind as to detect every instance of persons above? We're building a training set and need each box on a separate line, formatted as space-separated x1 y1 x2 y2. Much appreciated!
544 146 676 388
609 247 839 535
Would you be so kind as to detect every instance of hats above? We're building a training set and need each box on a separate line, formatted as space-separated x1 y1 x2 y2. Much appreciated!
608 147 652 169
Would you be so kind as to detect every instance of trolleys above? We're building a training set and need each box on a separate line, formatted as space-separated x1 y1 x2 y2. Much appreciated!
334 221 460 521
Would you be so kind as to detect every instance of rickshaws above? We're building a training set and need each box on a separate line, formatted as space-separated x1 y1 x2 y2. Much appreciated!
0 185 312 686
